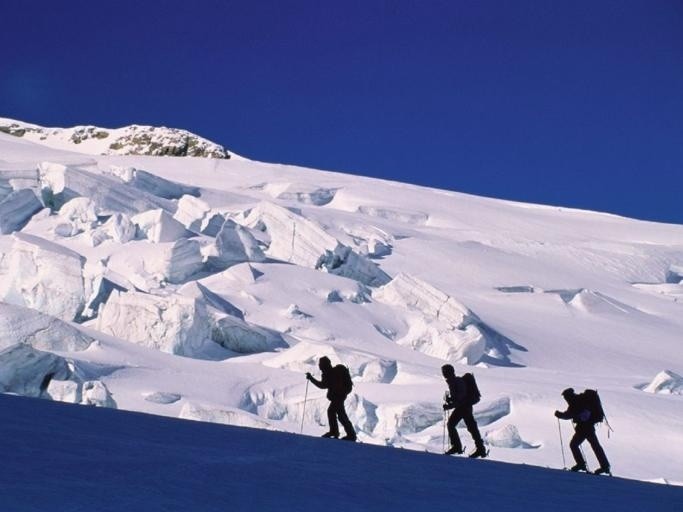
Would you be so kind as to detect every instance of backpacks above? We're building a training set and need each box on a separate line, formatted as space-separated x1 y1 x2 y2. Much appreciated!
463 373 480 404
334 364 352 393
583 389 603 423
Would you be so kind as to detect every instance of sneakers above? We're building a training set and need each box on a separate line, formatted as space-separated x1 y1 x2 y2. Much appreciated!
323 431 339 438
595 467 609 474
445 446 462 455
571 464 585 471
470 449 486 458
341 434 356 441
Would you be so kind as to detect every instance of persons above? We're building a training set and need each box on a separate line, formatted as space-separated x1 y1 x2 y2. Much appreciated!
304 355 356 442
440 364 487 458
553 387 610 475
